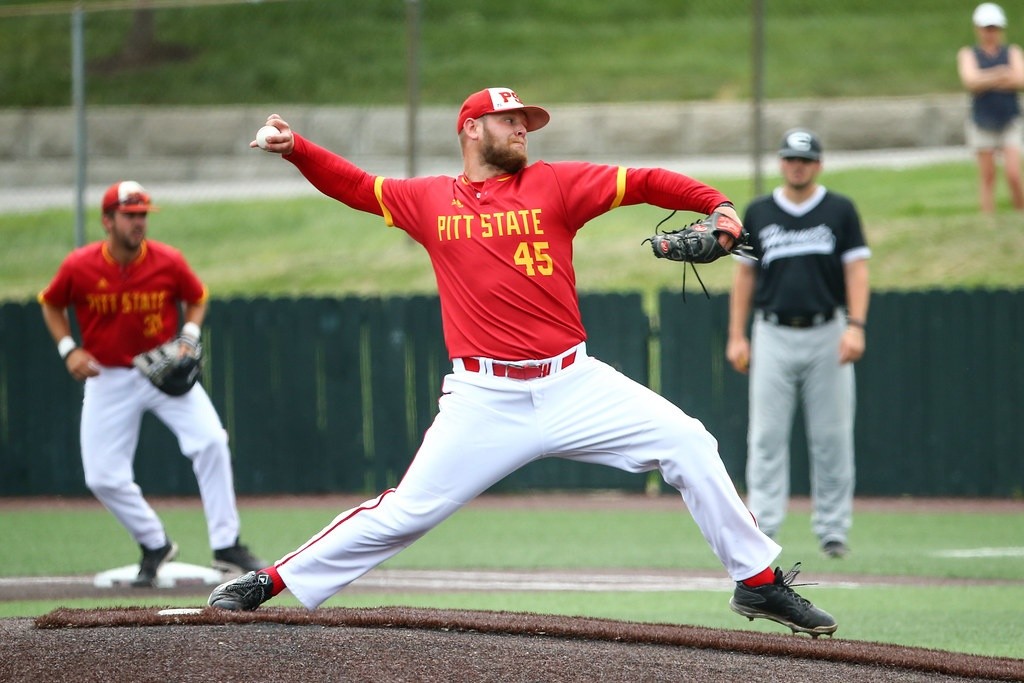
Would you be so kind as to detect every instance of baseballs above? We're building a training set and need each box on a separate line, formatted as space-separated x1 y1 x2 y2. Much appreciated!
256 125 281 151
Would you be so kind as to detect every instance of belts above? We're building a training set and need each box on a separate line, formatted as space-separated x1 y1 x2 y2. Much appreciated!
463 351 576 380
764 310 833 327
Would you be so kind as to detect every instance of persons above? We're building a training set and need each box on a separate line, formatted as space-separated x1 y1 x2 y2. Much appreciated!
956 2 1024 213
37 181 261 589
726 129 873 561
206 87 839 639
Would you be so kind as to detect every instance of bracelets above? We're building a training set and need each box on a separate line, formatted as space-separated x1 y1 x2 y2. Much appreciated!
184 321 203 339
56 334 77 359
846 319 865 330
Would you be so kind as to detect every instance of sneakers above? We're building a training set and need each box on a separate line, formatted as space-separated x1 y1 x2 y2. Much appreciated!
208 570 275 611
729 561 837 638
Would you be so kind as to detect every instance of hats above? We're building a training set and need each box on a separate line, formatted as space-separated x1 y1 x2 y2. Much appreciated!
457 87 550 136
779 129 822 161
973 3 1007 27
103 181 157 212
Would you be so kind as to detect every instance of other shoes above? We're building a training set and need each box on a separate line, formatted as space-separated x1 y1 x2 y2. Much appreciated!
133 543 176 587
212 537 266 574
823 541 847 557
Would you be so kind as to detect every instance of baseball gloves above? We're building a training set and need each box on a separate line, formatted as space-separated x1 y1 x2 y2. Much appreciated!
650 211 749 264
131 336 203 396
